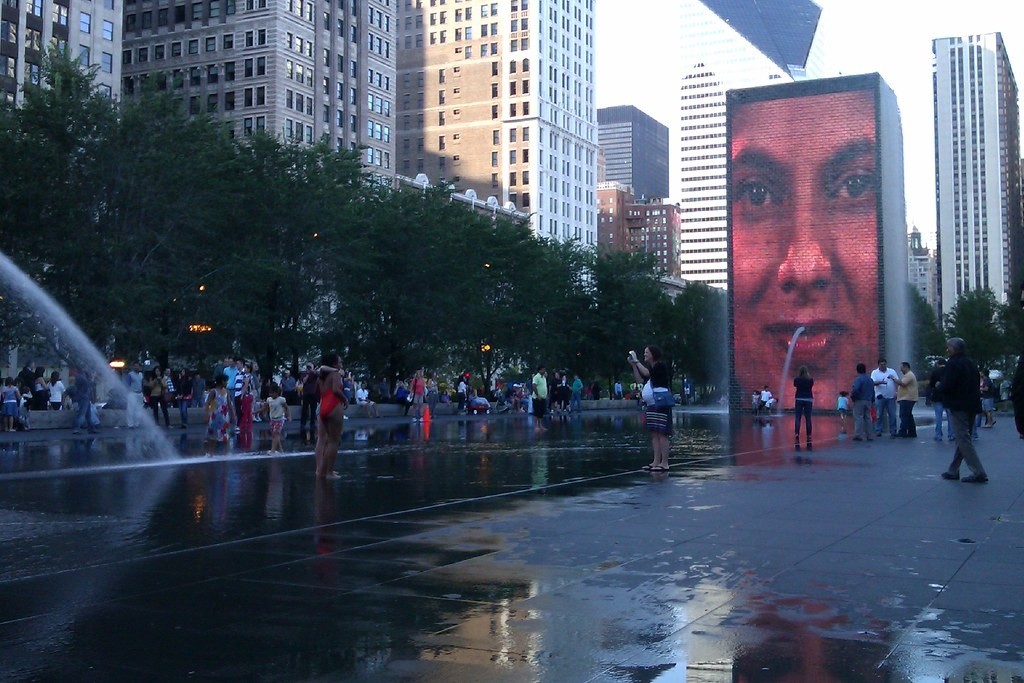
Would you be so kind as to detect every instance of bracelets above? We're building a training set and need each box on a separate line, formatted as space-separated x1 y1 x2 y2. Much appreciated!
635 360 639 363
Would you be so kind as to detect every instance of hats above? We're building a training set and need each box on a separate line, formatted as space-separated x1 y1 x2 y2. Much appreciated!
285 370 290 373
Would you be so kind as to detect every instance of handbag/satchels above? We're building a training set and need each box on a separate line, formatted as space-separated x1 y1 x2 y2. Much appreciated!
89 401 100 426
163 392 171 402
406 393 415 406
653 391 675 409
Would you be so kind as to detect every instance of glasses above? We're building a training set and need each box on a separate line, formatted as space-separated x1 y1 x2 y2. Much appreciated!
1020 301 1024 307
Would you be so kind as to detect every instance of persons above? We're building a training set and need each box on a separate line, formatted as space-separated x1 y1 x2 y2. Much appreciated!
627 345 672 472
870 358 901 439
0 356 626 480
894 362 918 438
850 363 875 441
926 358 956 441
976 368 997 428
1000 380 1008 412
794 366 814 451
836 391 850 434
933 337 988 484
732 89 878 412
752 386 779 416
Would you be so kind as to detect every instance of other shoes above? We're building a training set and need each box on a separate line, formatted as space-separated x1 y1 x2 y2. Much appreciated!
179 422 187 429
235 428 239 431
792 420 997 444
9 429 16 432
168 426 174 429
961 475 989 482
128 424 135 428
253 417 262 422
89 429 100 435
942 472 959 478
73 431 82 433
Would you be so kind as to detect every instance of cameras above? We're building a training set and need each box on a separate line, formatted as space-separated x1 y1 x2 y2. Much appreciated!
629 355 633 361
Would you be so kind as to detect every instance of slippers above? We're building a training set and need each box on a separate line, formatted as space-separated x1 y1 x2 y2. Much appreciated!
644 464 653 470
650 466 670 471
